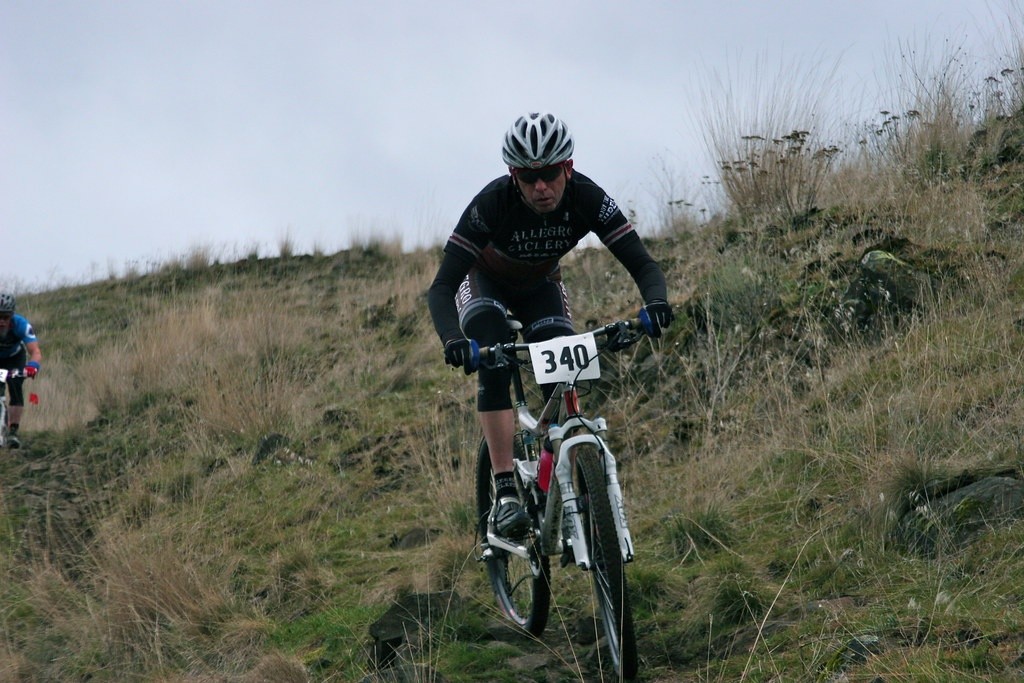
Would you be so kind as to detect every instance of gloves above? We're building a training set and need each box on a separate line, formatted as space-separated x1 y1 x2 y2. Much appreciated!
444 339 479 376
637 300 672 338
23 365 38 378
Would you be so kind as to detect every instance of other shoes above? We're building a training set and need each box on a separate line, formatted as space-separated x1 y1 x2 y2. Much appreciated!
7 433 20 449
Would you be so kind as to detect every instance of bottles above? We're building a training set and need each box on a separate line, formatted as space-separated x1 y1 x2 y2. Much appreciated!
537 436 554 493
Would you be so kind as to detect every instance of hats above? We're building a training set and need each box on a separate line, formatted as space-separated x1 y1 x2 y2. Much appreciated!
0 293 16 311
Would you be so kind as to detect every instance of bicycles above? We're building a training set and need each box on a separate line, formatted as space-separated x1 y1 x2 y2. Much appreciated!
440 308 678 683
0 368 34 451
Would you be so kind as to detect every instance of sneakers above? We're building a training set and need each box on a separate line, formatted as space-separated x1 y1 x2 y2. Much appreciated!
492 494 532 539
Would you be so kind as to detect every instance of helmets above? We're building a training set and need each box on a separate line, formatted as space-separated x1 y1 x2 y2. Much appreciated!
501 112 574 170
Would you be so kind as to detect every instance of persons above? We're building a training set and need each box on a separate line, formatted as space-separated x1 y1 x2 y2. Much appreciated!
0 292 42 450
427 111 677 567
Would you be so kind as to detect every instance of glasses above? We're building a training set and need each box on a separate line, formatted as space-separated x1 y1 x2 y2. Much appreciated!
512 159 569 184
0 312 13 321
